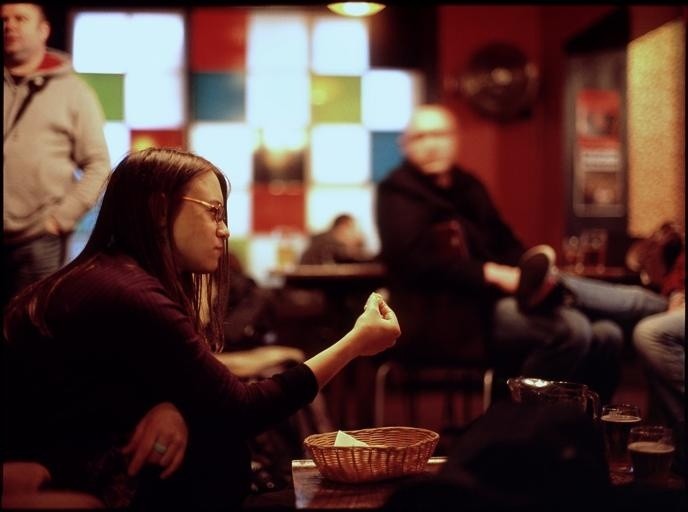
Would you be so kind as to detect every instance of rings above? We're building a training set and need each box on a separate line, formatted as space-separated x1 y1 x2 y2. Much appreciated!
153 441 167 456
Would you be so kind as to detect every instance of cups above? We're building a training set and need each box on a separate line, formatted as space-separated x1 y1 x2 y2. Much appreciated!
600 402 675 488
506 375 600 425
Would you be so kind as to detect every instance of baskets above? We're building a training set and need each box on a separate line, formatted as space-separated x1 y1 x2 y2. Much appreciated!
304 427 440 482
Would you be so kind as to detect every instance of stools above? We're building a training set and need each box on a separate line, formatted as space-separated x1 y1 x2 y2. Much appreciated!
368 363 493 447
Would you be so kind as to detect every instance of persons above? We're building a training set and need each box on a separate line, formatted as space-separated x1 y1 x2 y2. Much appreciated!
517 244 687 436
300 216 364 265
2 148 402 508
2 3 111 297
378 106 623 436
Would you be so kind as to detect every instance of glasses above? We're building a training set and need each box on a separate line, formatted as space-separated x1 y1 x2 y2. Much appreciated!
181 196 226 223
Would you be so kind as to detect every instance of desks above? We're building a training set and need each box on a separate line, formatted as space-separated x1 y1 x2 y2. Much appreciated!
8 454 685 510
278 262 383 307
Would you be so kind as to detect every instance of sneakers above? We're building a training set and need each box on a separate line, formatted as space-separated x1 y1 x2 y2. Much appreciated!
513 246 559 307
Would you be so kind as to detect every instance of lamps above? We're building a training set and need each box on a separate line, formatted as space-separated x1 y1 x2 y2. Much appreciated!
327 0 388 20
